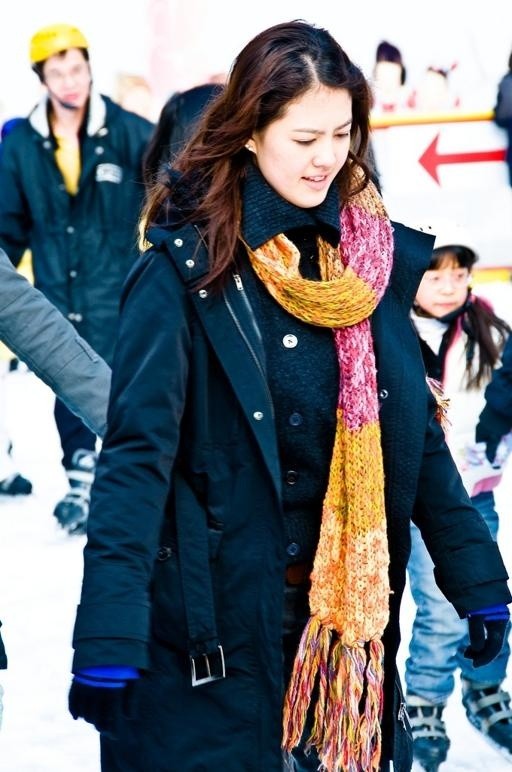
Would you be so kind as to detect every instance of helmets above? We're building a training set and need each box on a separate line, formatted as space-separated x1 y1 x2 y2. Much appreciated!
413 221 480 263
31 25 84 65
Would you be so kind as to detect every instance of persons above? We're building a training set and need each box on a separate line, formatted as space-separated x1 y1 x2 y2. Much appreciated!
0 21 162 529
66 16 512 771
141 76 225 203
398 225 512 764
357 28 421 113
0 244 114 448
489 50 510 189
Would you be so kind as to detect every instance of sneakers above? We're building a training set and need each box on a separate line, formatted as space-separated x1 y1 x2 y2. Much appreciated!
459 676 512 745
407 705 448 762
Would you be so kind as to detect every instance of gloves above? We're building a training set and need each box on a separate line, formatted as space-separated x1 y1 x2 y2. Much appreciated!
68 666 137 736
463 606 508 667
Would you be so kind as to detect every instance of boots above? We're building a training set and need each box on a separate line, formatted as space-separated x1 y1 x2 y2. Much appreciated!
55 446 100 527
